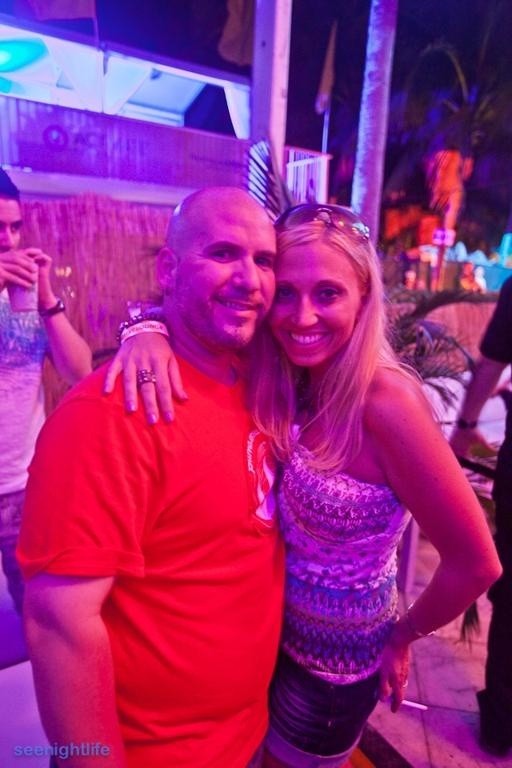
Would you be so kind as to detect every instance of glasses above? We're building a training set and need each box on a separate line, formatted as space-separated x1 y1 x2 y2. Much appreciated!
275 204 370 241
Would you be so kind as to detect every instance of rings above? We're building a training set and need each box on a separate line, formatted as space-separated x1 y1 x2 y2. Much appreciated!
137 368 155 387
403 679 409 689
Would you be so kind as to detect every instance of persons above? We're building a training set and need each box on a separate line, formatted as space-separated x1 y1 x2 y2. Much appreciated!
104 204 506 767
15 186 289 767
449 276 512 754
0 167 94 672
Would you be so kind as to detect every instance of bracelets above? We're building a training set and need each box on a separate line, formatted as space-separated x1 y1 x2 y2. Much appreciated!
119 319 172 343
37 296 67 318
454 413 480 429
404 600 429 639
116 307 166 344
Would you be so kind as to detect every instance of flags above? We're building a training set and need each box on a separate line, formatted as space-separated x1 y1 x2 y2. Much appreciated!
28 1 96 22
314 21 337 116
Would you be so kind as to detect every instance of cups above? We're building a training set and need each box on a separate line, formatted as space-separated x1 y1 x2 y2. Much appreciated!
6 262 39 311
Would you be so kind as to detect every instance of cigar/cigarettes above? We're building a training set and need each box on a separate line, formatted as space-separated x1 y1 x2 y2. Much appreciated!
401 699 428 711
